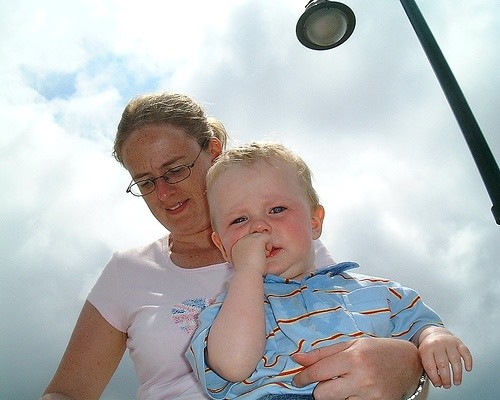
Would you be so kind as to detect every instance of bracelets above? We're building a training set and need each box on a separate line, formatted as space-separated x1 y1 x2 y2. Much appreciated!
404 372 428 400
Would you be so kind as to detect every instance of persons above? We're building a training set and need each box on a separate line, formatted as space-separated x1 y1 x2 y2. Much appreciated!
185 140 473 400
38 91 430 400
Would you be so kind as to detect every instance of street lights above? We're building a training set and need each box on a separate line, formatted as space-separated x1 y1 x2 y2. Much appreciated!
295 0 499 227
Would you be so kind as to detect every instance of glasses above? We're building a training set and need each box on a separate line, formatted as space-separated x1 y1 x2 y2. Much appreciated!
126 134 211 197
